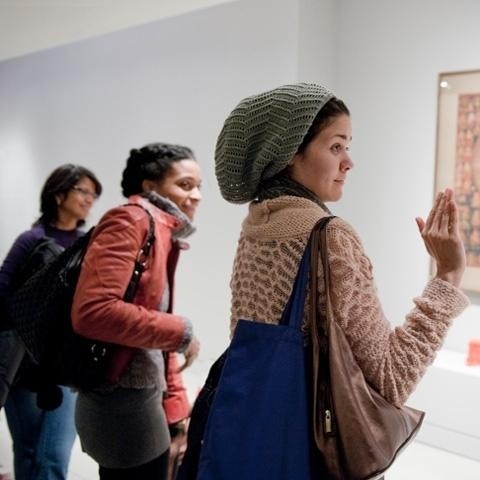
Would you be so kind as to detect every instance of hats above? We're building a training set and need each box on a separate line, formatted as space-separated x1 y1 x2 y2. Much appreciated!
214 81 336 204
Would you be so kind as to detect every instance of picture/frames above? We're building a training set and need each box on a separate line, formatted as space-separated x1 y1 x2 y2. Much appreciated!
429 67 480 294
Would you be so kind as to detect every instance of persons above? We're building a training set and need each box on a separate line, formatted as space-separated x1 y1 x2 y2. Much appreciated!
74 143 200 480
230 81 466 479
0 162 102 480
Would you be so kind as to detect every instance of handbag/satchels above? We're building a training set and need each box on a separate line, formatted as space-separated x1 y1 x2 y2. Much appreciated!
197 319 311 479
3 226 145 392
313 321 425 479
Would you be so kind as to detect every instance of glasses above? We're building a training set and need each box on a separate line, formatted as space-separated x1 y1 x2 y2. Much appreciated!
72 185 98 200
